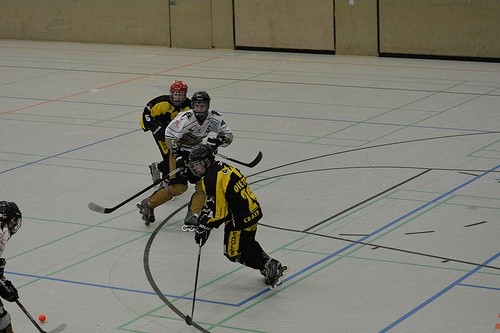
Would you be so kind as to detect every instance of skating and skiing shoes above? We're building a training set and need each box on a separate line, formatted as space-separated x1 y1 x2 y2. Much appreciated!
149 162 160 182
260 256 289 290
136 198 155 226
182 210 198 232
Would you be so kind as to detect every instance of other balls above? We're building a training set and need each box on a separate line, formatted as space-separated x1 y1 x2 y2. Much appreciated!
39 314 46 322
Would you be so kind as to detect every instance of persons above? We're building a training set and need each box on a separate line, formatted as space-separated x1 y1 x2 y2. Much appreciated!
137 91 233 231
0 201 22 333
189 143 288 291
140 81 192 187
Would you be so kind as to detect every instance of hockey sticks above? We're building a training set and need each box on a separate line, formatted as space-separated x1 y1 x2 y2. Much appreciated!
185 238 202 325
88 167 182 215
215 151 263 168
15 299 47 333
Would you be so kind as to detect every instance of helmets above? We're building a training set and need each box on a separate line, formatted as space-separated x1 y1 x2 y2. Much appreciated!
189 143 215 178
170 80 188 106
0 201 22 240
192 91 211 116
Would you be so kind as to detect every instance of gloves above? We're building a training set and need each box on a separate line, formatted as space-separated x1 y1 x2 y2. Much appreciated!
176 156 187 174
207 137 222 148
198 205 211 222
0 276 19 302
152 124 165 142
195 221 213 247
